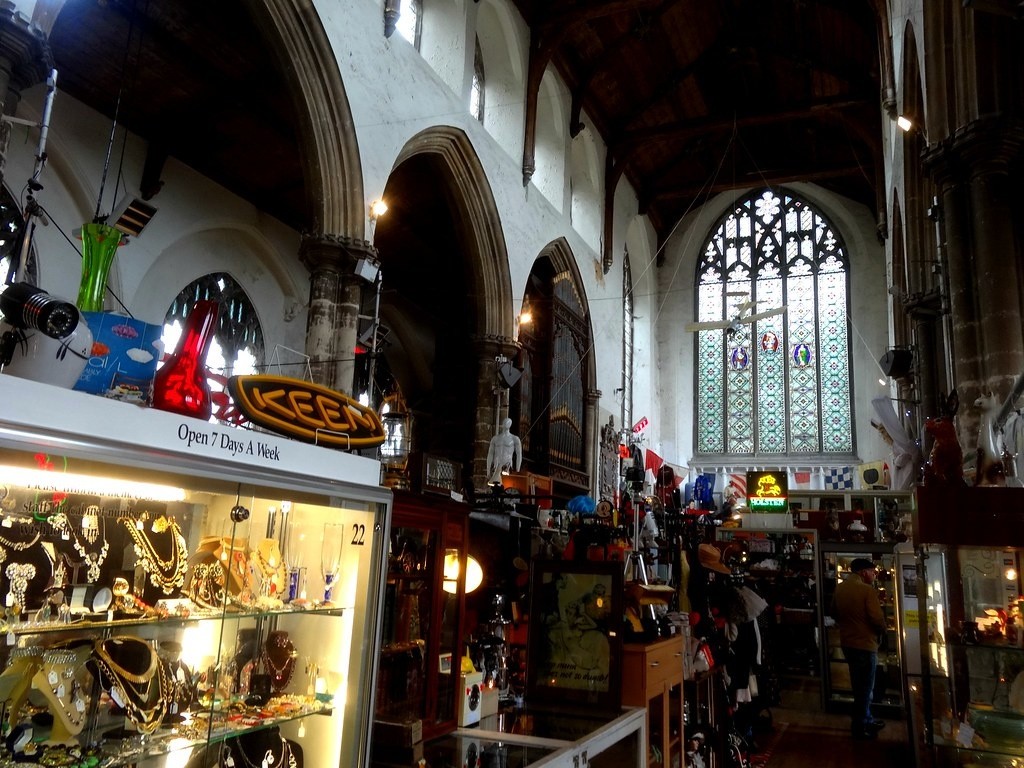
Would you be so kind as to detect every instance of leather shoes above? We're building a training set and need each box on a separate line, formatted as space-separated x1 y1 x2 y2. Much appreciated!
852 730 878 740
865 720 885 732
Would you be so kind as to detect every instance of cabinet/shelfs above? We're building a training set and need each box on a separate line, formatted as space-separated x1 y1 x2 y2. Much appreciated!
788 490 916 714
0 371 395 768
619 634 685 768
915 487 1024 768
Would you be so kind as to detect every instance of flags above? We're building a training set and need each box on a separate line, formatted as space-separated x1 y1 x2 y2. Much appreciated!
824 465 851 490
730 474 747 498
858 459 885 490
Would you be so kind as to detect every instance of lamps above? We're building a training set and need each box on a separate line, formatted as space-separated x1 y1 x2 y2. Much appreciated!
377 381 414 490
502 461 512 476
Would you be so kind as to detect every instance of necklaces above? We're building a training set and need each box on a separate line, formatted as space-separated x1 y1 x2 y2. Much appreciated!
0 505 296 768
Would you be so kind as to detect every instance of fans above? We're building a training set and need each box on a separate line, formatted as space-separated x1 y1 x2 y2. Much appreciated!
684 297 789 334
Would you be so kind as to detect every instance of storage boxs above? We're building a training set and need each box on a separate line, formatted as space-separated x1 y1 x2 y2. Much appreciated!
501 472 553 509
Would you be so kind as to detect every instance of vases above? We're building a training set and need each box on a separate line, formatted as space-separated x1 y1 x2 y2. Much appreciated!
76 223 121 312
153 300 224 420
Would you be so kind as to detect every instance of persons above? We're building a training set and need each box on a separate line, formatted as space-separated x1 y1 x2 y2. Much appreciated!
830 558 887 738
531 573 609 680
486 418 522 483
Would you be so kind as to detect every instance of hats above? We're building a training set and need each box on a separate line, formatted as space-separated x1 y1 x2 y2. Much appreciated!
698 544 731 574
850 558 876 573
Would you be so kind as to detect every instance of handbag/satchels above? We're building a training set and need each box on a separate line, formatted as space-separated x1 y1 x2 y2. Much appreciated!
621 445 784 768
